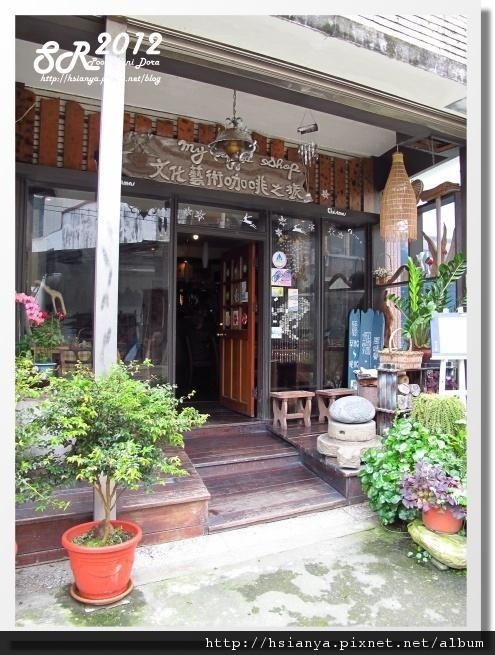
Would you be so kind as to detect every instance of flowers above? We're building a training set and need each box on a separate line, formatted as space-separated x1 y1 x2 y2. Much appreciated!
373 265 394 279
398 463 466 518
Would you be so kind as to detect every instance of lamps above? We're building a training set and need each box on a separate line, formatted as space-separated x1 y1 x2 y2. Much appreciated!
208 90 257 162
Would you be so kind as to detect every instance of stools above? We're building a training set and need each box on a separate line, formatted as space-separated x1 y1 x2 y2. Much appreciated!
270 388 353 432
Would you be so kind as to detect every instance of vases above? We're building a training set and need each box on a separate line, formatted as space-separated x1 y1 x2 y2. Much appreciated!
421 501 464 533
379 277 386 285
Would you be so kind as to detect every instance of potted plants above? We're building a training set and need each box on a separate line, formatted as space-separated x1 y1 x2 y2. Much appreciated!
28 359 211 608
387 253 466 360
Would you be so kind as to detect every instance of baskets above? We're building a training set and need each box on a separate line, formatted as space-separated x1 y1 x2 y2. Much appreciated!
378 328 424 371
